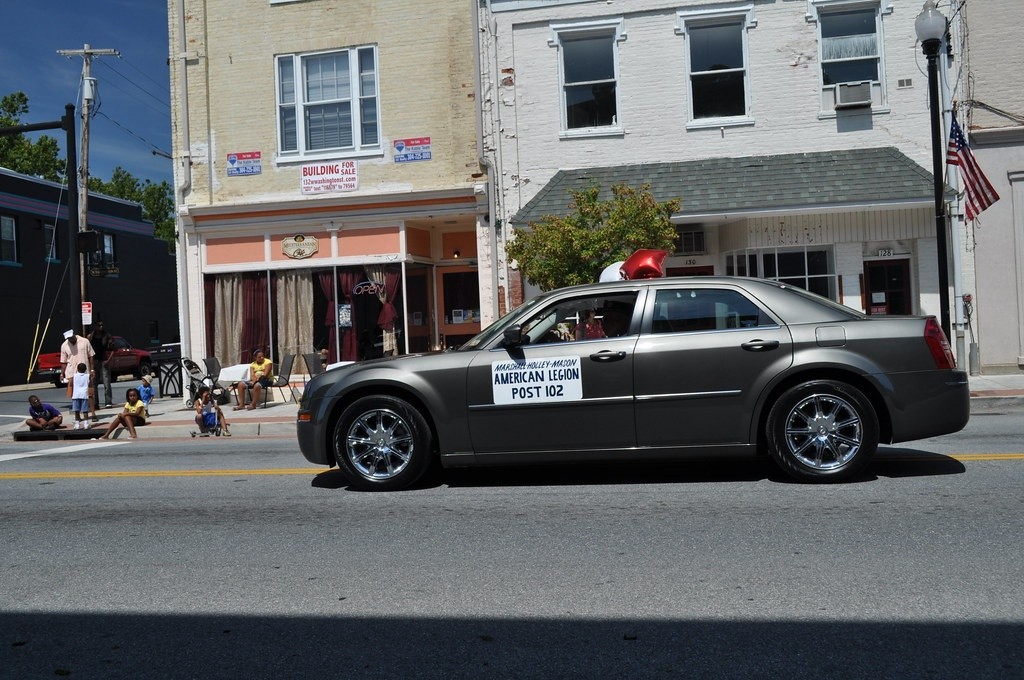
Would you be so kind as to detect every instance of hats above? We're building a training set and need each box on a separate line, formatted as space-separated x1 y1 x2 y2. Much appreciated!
63 329 73 339
142 375 153 386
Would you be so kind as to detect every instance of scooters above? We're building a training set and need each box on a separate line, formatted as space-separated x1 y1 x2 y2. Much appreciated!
191 382 224 438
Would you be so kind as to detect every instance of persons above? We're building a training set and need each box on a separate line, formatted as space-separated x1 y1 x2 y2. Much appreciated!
233 346 273 410
26 395 62 431
576 310 605 341
70 363 93 430
98 388 146 440
137 375 154 417
60 321 114 421
195 387 231 436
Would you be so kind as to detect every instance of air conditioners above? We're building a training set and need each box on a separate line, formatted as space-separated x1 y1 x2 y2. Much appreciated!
833 79 874 110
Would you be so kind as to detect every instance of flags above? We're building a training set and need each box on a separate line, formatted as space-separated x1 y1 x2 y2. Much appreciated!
947 111 999 226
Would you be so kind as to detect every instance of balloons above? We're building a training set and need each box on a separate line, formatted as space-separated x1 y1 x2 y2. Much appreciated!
618 248 670 279
599 261 626 283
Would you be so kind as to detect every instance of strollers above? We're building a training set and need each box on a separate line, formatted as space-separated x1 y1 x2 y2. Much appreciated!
178 356 218 408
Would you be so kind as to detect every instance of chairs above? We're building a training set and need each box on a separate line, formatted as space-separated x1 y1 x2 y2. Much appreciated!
250 354 298 408
303 352 325 378
201 357 253 405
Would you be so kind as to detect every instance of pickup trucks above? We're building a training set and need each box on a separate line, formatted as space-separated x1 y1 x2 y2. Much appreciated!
38 335 153 387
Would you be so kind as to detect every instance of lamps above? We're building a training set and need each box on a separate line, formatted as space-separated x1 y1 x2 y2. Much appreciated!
454 251 460 258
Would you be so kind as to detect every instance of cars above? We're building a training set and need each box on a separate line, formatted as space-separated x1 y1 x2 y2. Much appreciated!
296 249 972 492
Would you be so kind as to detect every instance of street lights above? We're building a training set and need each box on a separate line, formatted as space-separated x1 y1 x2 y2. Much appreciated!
915 0 951 346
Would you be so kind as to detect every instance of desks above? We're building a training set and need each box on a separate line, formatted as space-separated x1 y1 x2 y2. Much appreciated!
219 363 253 406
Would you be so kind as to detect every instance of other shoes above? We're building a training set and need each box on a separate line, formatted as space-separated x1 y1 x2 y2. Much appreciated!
99 437 109 441
145 413 149 418
30 426 34 431
247 403 256 410
235 404 245 410
74 424 80 430
92 416 99 422
49 424 55 430
106 403 112 408
84 423 91 429
127 436 137 441
223 429 231 436
94 405 99 409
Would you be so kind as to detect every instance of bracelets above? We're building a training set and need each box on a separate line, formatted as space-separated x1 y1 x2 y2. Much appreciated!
60 374 63 376
199 406 203 409
91 370 95 372
59 415 62 417
